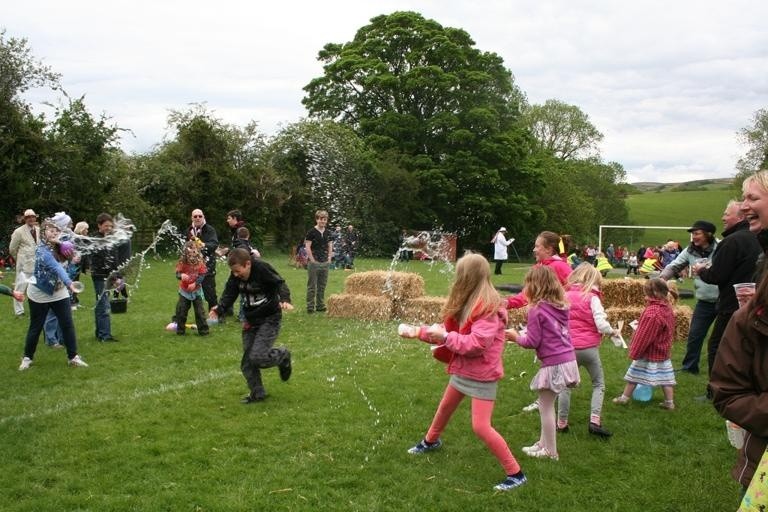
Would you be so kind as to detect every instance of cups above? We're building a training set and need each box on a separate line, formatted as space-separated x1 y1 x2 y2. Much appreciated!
398 323 414 338
505 327 529 343
427 323 442 333
609 336 622 347
733 283 756 310
628 318 639 331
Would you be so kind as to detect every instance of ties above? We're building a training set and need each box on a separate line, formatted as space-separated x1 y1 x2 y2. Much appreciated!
31 230 37 242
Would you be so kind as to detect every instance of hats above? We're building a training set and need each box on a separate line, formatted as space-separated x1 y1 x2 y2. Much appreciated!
687 221 716 233
21 209 39 220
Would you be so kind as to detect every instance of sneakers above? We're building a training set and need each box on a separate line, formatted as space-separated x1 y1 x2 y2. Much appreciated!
521 400 540 411
407 439 442 455
659 402 675 410
19 356 33 372
492 475 528 492
556 425 569 433
279 350 291 381
612 397 631 406
240 394 265 403
522 441 559 462
307 307 327 314
98 337 121 344
589 422 613 437
68 355 89 367
47 343 64 350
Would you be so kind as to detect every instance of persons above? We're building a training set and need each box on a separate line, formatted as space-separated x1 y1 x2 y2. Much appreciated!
612 278 681 410
399 229 410 263
287 219 356 270
305 208 334 314
497 231 576 412
490 226 516 276
657 221 721 374
565 238 691 282
1 208 260 345
397 252 528 492
504 264 582 462
208 247 296 404
16 219 90 371
555 260 622 439
706 255 767 512
692 200 762 402
738 169 767 287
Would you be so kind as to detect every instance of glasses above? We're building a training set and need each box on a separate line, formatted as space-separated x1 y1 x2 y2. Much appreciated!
192 215 204 218
